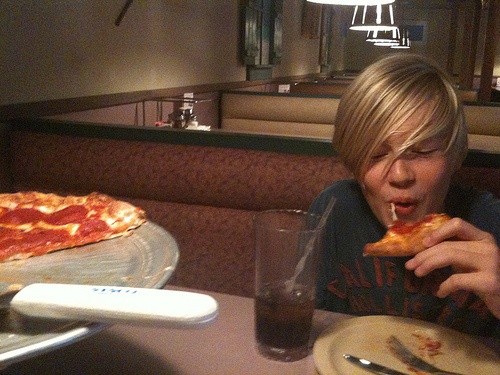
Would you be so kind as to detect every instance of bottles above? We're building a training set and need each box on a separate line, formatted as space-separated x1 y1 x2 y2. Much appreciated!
174 108 198 130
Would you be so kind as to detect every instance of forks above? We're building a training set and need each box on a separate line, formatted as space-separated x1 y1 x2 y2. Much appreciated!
387 335 466 375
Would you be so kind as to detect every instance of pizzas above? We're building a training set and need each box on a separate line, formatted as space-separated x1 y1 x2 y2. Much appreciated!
0 191 145 262
363 212 452 257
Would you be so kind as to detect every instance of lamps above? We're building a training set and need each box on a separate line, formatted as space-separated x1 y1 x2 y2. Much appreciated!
306 0 411 49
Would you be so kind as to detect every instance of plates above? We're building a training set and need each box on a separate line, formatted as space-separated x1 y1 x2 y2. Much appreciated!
0 221 179 367
313 314 500 375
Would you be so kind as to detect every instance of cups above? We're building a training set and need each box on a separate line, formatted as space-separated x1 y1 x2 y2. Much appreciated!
252 209 327 361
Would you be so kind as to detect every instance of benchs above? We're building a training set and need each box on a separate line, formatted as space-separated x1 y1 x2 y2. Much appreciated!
220 89 500 153
0 117 500 298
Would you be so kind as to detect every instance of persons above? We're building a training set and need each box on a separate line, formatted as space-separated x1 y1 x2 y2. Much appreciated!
298 53 500 338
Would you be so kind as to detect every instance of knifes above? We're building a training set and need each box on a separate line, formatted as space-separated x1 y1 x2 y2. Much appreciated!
342 353 409 375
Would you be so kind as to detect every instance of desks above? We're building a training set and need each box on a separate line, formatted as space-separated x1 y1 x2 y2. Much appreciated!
0 284 357 375
